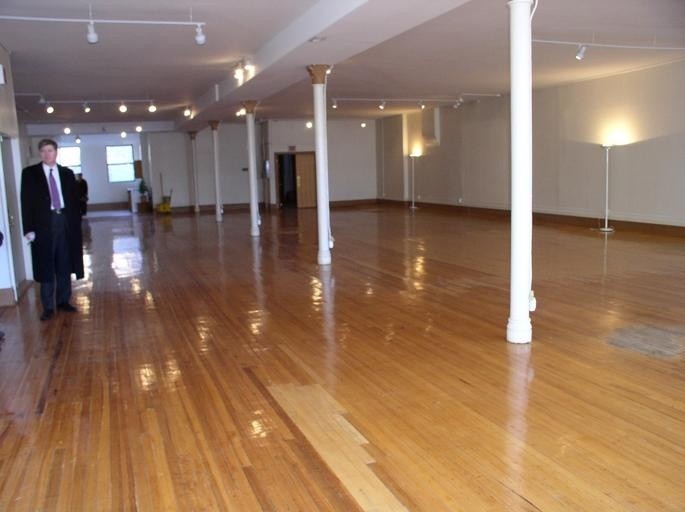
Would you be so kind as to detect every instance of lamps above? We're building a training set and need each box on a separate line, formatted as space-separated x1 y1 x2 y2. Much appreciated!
0 0 208 46
331 99 463 110
600 143 615 232
408 154 421 208
183 104 192 117
234 56 253 78
534 41 683 60
38 99 156 144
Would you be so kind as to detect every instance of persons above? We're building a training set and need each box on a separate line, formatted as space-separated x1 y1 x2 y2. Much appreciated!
19 138 86 322
75 172 88 218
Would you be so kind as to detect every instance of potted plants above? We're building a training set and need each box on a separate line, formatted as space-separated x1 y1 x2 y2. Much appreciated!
138 179 147 202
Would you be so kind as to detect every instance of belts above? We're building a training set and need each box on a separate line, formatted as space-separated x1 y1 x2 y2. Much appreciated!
50 208 66 214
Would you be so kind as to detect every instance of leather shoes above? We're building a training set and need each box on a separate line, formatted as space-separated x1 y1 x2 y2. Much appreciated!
39 300 77 321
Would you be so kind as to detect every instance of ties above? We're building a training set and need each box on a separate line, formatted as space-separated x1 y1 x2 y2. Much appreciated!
48 168 62 208
